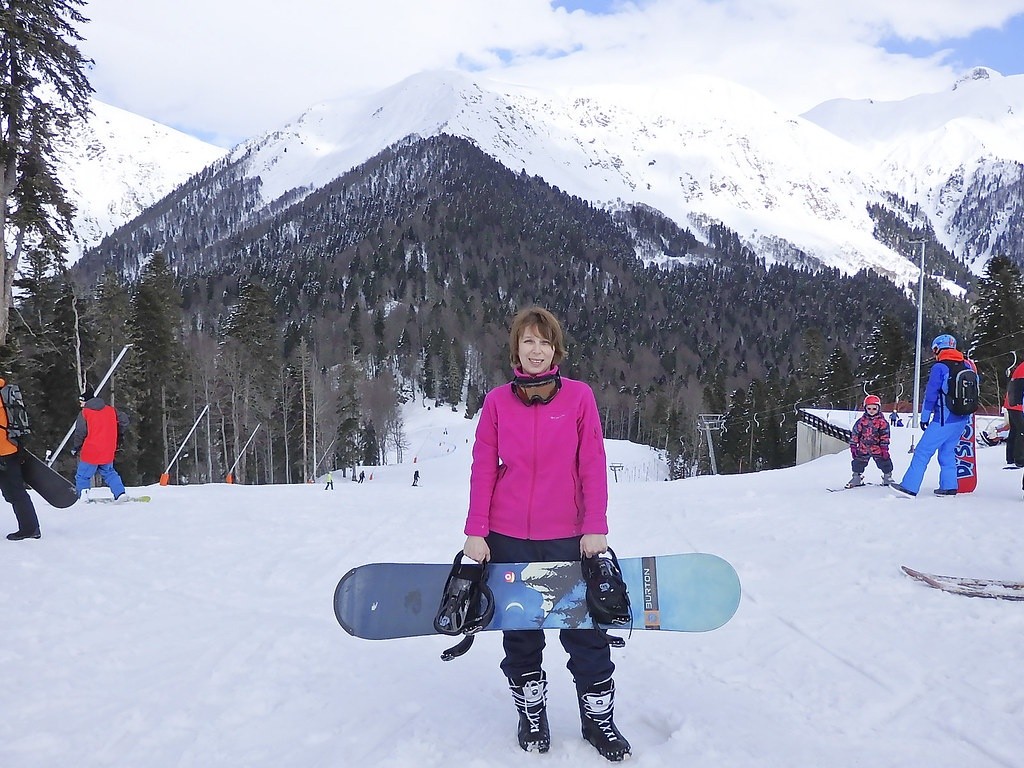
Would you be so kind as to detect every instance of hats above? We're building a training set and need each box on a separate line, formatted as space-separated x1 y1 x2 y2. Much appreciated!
79 393 94 402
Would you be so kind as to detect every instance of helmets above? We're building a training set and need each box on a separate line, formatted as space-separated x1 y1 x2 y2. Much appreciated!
932 335 957 355
863 395 881 407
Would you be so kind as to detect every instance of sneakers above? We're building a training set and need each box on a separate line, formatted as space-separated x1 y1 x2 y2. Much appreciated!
573 677 633 763
888 483 916 500
930 489 958 497
78 489 93 503
882 472 895 485
111 494 128 502
7 528 41 540
509 670 550 752
844 472 865 488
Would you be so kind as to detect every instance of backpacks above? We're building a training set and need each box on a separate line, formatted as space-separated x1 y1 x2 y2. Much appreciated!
0 384 32 448
933 360 978 415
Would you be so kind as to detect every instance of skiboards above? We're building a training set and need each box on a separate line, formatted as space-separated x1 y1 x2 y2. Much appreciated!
826 472 898 493
900 564 1024 602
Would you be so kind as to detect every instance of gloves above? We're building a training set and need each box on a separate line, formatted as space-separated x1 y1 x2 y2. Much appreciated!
851 445 858 459
920 421 929 431
880 446 890 460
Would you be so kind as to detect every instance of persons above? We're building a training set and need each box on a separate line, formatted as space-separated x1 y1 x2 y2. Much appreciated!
358 470 365 484
847 395 895 487
69 388 128 505
462 307 633 764
976 361 1024 468
889 409 904 427
889 334 972 500
411 470 420 487
0 374 41 541
324 471 334 490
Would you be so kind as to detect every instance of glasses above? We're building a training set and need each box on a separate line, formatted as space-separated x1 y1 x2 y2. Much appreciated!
512 376 562 407
867 408 876 410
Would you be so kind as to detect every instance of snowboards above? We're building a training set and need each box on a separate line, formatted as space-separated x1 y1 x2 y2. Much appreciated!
332 550 741 642
22 446 78 508
